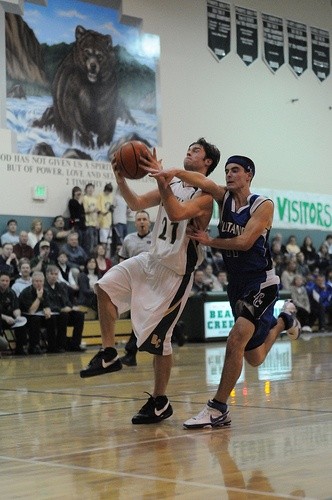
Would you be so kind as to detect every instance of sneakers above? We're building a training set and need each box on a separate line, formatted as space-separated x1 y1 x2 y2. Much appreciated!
281 301 301 340
131 390 173 424
80 347 123 378
184 399 232 429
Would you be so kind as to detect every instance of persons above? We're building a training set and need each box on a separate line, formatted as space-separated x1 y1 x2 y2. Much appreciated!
80 138 221 424
191 252 228 292
143 155 301 429
68 183 130 258
0 215 112 356
271 233 332 332
118 211 187 367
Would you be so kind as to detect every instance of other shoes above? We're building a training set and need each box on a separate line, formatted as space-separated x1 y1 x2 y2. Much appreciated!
14 338 82 354
121 351 138 367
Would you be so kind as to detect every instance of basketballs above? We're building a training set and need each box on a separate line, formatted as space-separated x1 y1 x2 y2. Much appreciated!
113 139 152 180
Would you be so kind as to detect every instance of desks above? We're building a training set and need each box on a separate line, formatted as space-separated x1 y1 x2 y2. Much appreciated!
189 290 292 343
